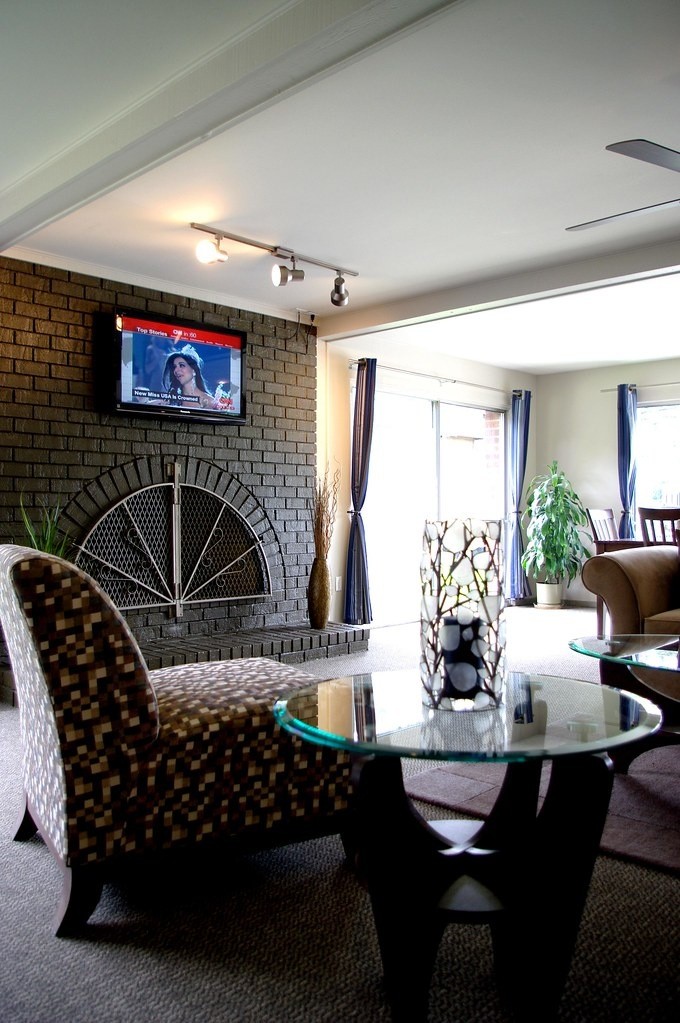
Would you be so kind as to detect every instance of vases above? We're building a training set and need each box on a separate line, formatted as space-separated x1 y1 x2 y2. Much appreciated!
307 558 330 630
420 518 509 711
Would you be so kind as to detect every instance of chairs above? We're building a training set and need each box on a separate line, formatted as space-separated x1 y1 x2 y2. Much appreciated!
637 506 680 550
585 506 623 543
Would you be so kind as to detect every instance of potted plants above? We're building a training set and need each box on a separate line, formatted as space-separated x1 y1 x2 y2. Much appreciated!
522 460 592 612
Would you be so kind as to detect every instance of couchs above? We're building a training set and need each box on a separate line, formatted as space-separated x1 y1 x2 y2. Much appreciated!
581 545 680 654
0 543 378 936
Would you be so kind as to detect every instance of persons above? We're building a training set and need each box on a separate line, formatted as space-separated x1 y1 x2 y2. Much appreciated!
143 344 225 414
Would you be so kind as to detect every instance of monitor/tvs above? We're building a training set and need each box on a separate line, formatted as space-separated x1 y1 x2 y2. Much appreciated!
110 307 248 426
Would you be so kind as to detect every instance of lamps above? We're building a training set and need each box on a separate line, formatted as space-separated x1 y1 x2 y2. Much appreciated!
271 256 306 288
331 271 351 307
196 232 229 265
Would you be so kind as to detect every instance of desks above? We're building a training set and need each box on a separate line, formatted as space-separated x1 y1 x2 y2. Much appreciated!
569 634 680 774
269 665 620 1023
592 540 680 640
